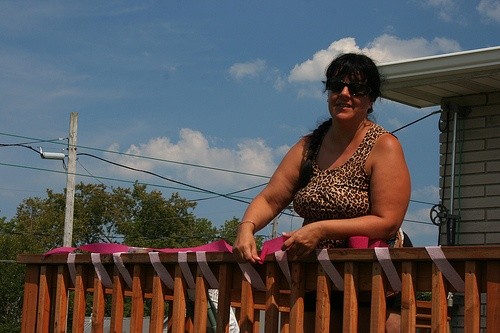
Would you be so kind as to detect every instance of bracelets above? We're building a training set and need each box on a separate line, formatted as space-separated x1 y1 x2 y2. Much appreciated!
238 220 257 232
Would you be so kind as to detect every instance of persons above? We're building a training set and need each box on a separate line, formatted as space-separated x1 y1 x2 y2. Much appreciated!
233 52 416 333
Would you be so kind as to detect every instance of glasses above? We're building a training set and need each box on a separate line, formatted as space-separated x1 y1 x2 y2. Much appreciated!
326 79 372 97
